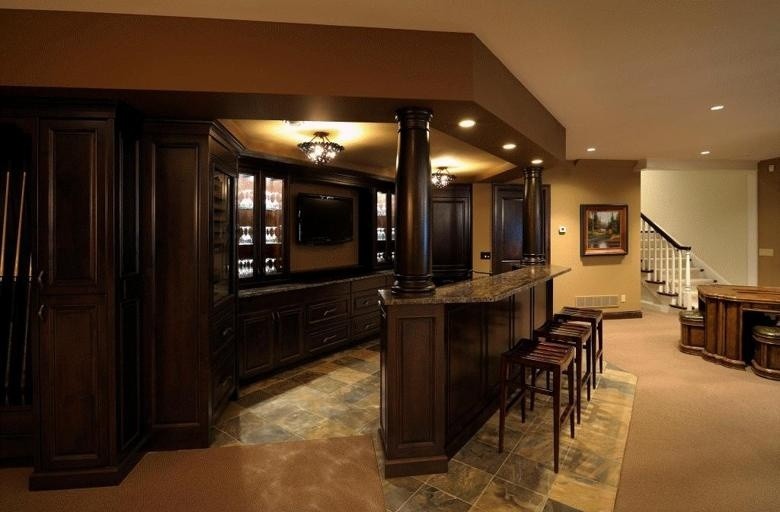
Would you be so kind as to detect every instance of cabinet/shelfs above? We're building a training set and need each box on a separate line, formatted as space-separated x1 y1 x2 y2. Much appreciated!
24 100 150 493
149 118 247 448
237 275 395 383
360 175 396 270
231 151 290 290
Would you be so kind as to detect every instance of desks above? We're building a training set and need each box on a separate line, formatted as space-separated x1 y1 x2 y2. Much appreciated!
696 284 780 371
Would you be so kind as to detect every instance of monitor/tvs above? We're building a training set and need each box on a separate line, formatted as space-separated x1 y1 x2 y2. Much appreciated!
294 192 354 247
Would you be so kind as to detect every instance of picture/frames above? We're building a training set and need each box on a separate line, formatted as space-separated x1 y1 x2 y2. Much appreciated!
580 204 628 256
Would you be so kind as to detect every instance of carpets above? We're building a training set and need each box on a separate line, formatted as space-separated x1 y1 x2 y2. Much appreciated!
0 435 385 512
604 308 780 512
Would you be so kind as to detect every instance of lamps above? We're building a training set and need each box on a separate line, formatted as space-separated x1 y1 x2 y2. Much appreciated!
297 131 344 167
431 166 455 191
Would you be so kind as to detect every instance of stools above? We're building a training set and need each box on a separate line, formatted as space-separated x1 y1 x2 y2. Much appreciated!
498 305 604 473
678 309 704 355
751 324 780 380
